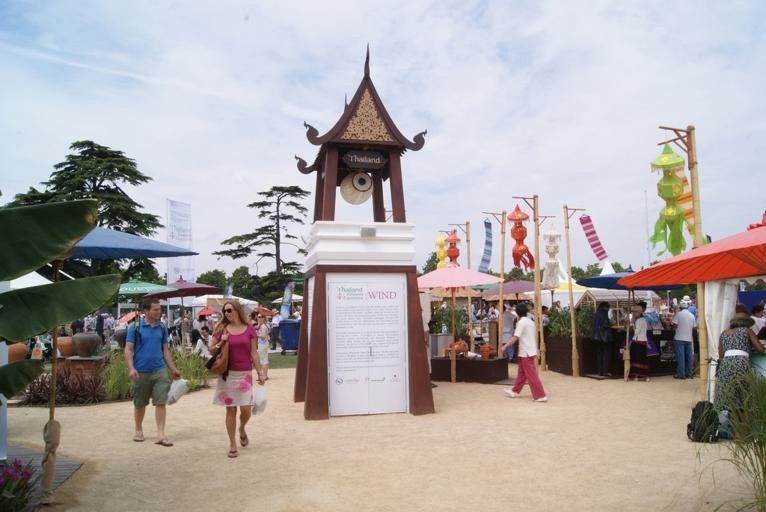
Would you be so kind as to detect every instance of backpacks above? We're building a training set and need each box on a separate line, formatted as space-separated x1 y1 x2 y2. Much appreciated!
687 402 722 443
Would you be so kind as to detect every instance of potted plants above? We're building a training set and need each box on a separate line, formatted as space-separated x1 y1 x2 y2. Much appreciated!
487 343 497 359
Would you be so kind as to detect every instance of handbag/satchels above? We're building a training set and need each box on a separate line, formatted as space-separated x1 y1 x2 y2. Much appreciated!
167 376 192 405
205 329 228 374
252 382 269 415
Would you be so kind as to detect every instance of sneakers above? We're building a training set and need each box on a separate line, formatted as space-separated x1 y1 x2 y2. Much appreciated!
504 389 517 397
532 396 548 401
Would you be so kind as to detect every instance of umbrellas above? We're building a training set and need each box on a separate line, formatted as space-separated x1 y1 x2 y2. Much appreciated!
619 219 761 288
416 262 505 382
142 275 223 320
117 278 179 296
49 227 200 419
475 276 555 304
576 264 686 299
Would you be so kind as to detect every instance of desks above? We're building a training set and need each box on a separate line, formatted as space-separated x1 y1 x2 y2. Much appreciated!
55 356 69 378
430 355 509 384
68 357 105 383
647 328 702 365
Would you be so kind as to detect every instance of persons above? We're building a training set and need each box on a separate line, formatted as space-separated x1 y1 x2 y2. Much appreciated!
124 298 301 458
64 311 115 338
484 301 550 402
441 301 447 308
629 295 697 378
591 302 613 376
712 299 766 410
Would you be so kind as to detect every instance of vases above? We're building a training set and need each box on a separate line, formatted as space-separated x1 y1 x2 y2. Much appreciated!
56 334 74 355
480 345 490 360
71 330 102 357
5 342 28 363
114 328 127 348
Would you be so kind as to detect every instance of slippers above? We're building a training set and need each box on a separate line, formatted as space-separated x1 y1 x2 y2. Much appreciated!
133 428 144 441
228 435 248 458
154 438 173 446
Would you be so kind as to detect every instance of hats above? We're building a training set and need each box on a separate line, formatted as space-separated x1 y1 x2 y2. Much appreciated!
680 295 692 308
730 312 755 327
630 306 643 312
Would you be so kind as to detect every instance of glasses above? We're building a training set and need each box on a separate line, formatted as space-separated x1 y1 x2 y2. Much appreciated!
223 309 231 313
